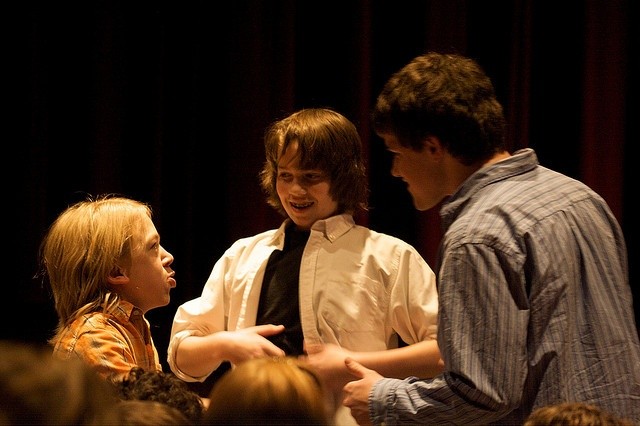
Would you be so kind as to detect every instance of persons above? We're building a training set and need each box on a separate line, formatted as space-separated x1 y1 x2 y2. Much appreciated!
0 340 122 426
523 402 636 426
343 51 640 426
111 367 208 426
34 194 177 384
167 109 445 426
201 356 337 426
